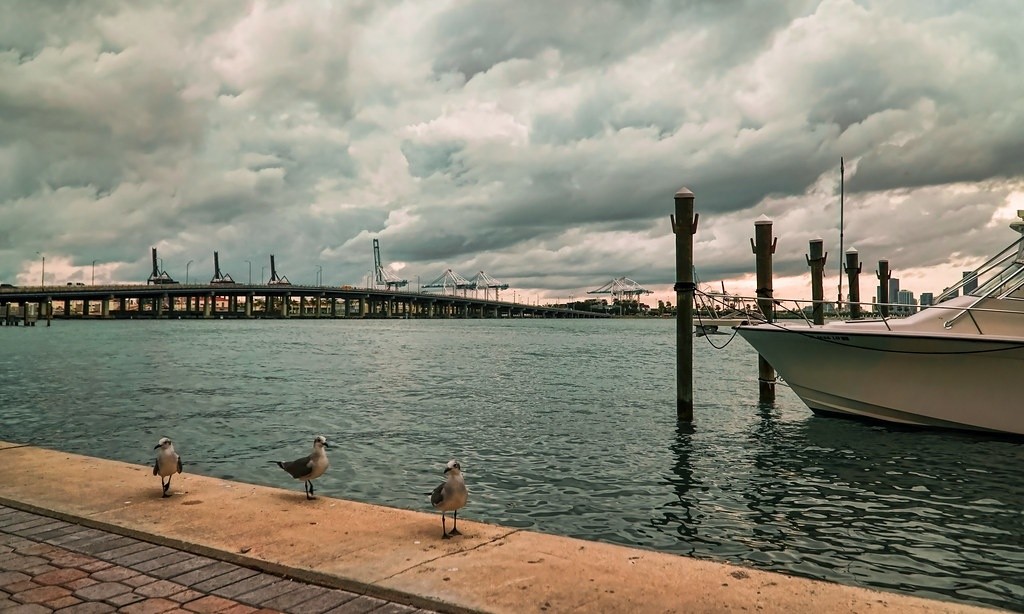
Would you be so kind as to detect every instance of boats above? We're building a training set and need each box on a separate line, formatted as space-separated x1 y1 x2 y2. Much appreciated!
730 209 1024 437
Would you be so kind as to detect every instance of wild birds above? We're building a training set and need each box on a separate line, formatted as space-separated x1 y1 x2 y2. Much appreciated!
430 459 469 540
265 436 329 500
152 437 183 498
